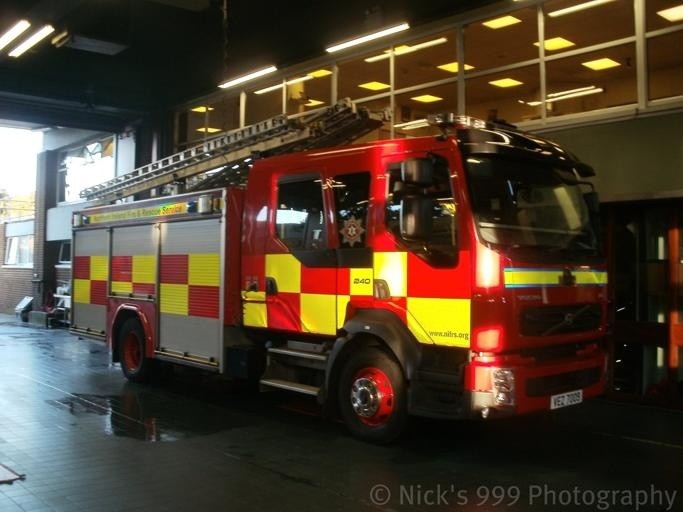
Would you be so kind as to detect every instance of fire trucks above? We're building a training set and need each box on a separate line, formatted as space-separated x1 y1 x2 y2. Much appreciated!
62 96 617 444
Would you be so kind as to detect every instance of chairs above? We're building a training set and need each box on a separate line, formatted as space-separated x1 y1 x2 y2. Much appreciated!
303 211 325 250
401 159 459 263
518 189 537 244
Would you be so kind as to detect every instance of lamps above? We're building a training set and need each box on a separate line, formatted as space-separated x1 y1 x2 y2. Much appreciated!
0 13 32 51
217 0 278 89
324 18 410 53
6 23 56 58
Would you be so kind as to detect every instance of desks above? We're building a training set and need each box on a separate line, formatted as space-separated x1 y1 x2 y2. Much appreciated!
53 294 72 320
